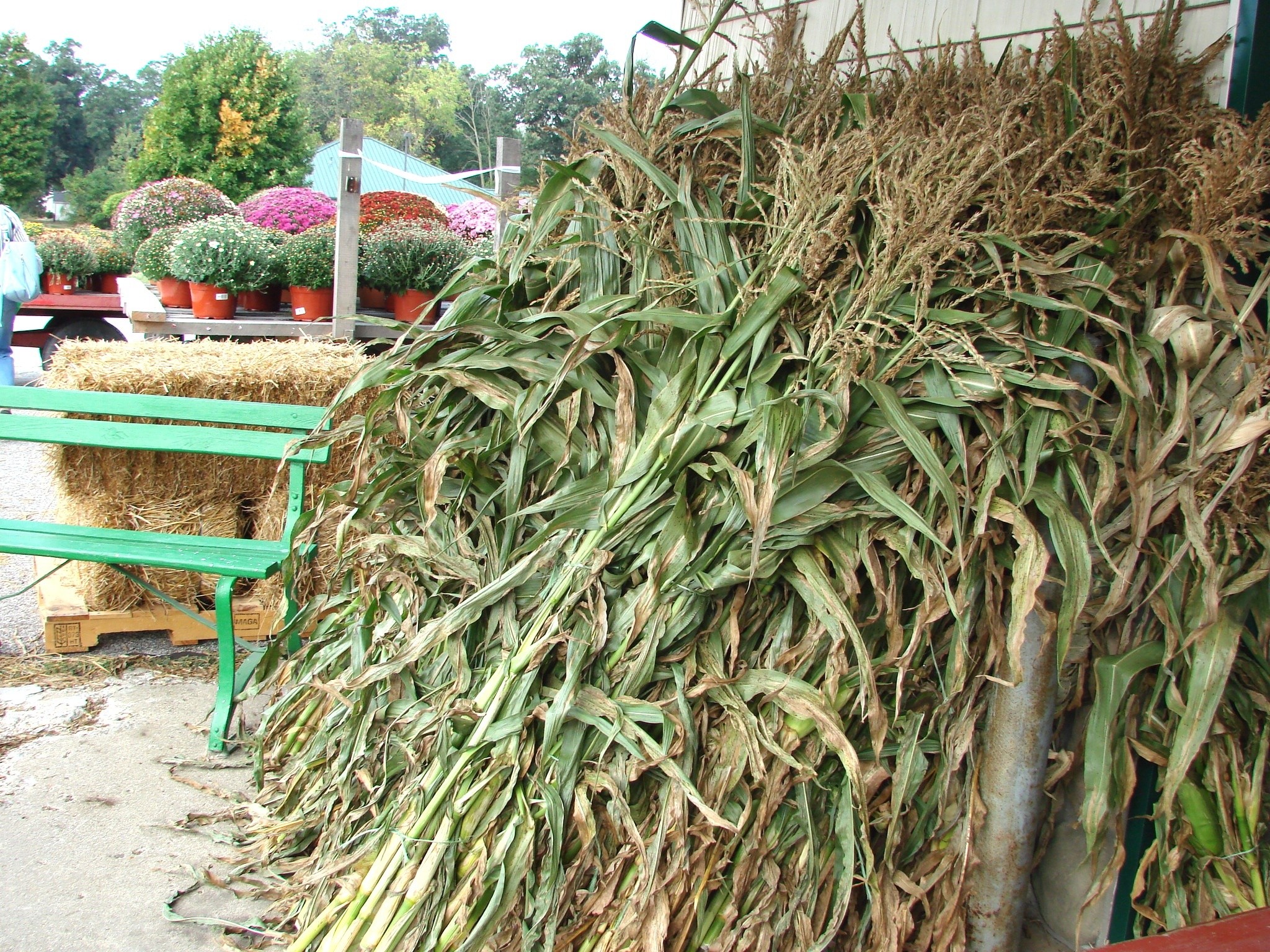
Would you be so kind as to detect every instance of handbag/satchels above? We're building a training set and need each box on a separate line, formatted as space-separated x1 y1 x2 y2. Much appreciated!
0 240 41 302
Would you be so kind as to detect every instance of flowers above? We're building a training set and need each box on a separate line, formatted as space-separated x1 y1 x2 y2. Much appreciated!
26 177 498 298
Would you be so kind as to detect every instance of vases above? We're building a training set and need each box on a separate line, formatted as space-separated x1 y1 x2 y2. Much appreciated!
43 272 466 324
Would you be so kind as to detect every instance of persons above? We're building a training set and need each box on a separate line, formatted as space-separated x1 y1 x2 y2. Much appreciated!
0 183 43 414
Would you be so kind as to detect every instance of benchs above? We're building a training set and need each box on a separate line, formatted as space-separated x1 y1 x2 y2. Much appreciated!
0 385 333 751
